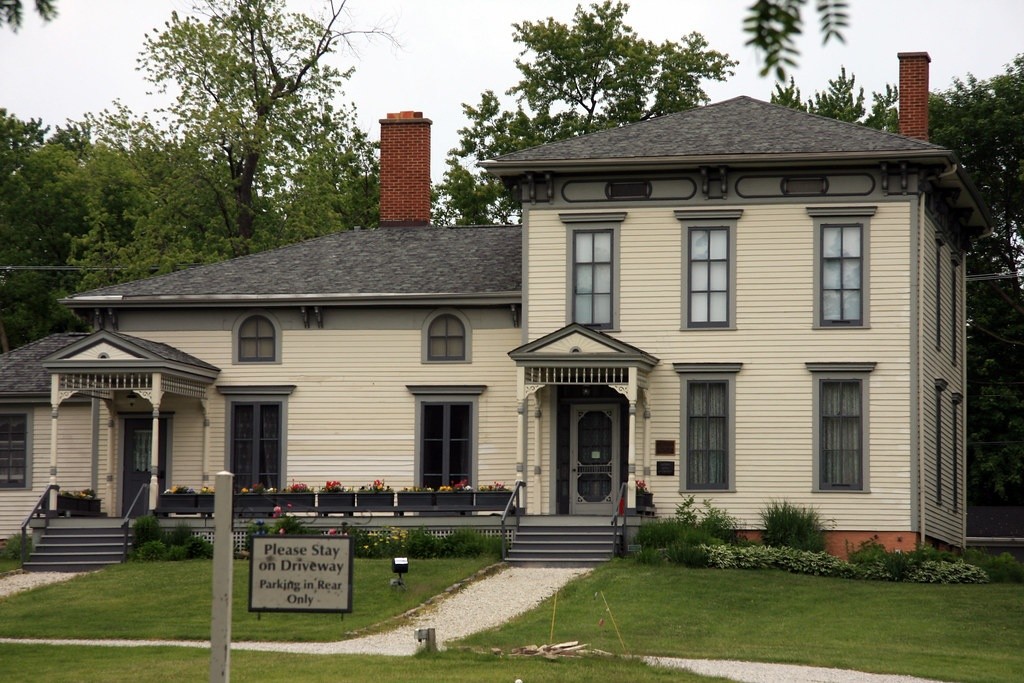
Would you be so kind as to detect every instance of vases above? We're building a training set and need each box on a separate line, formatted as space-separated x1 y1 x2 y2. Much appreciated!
435 491 473 505
57 496 102 513
636 494 653 506
475 491 513 506
198 494 215 508
235 493 316 507
317 492 357 507
396 490 434 506
160 494 198 507
357 492 395 506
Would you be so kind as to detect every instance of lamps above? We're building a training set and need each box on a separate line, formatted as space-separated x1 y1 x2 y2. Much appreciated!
583 386 590 400
126 390 139 407
391 557 409 574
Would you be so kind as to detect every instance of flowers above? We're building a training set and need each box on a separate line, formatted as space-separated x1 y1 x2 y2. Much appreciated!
200 486 215 494
439 479 474 494
164 484 198 495
318 481 355 492
476 481 510 491
240 483 277 496
61 488 96 500
403 486 434 493
358 480 394 496
636 480 650 494
282 479 315 493
